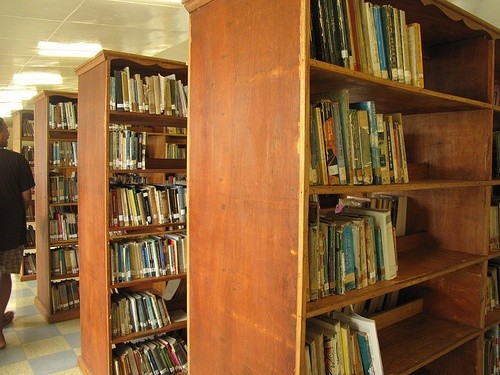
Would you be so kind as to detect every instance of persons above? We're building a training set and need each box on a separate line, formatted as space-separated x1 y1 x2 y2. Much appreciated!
0 117 36 328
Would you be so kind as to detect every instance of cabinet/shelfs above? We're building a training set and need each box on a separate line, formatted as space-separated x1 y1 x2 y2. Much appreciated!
12 110 36 282
181 1 500 375
32 90 80 325
74 50 189 375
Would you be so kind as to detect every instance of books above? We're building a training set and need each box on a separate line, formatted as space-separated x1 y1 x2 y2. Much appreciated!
49 101 79 313
485 83 500 375
109 124 186 169
109 173 188 228
306 195 401 303
309 0 424 89
112 278 188 338
22 118 36 274
310 90 408 186
109 67 188 118
304 290 399 375
110 226 188 285
112 335 187 375
